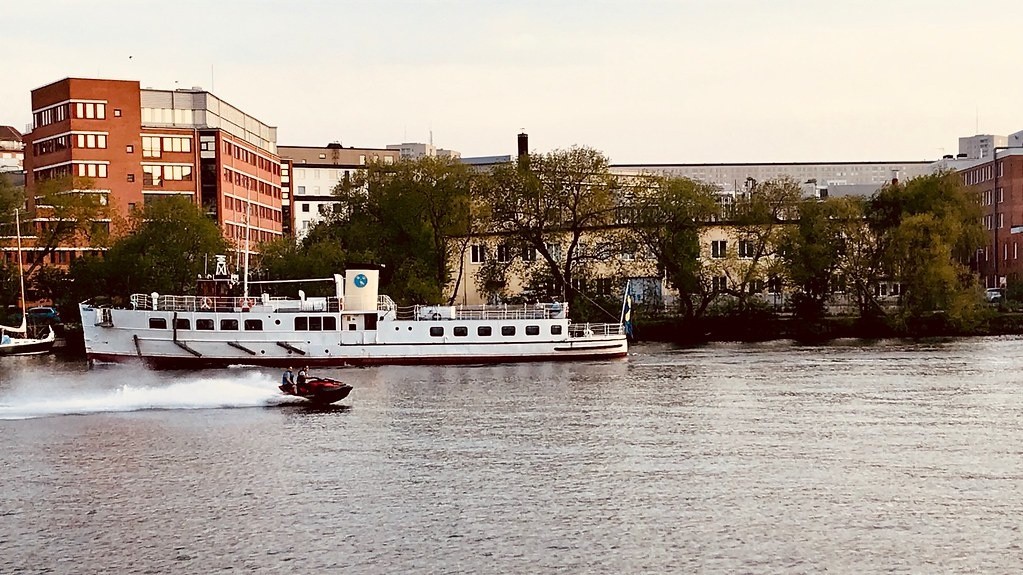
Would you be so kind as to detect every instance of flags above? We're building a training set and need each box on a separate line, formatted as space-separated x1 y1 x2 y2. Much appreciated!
625 284 632 337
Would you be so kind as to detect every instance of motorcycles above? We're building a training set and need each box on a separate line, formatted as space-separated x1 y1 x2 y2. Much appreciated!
278 376 354 404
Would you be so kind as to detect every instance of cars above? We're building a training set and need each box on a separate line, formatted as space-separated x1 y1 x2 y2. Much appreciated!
15 307 62 325
984 287 1004 303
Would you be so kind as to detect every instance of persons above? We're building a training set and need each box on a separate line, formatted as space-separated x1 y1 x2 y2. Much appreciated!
549 297 560 318
297 365 318 396
283 365 297 394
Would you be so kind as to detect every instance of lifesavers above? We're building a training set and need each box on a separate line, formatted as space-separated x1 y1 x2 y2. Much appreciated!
239 298 253 309
201 297 212 310
431 313 442 320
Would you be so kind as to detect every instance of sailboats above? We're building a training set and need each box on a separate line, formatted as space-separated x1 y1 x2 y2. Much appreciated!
0 208 56 357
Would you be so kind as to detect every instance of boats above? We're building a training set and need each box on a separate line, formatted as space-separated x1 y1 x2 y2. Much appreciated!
77 201 630 371
